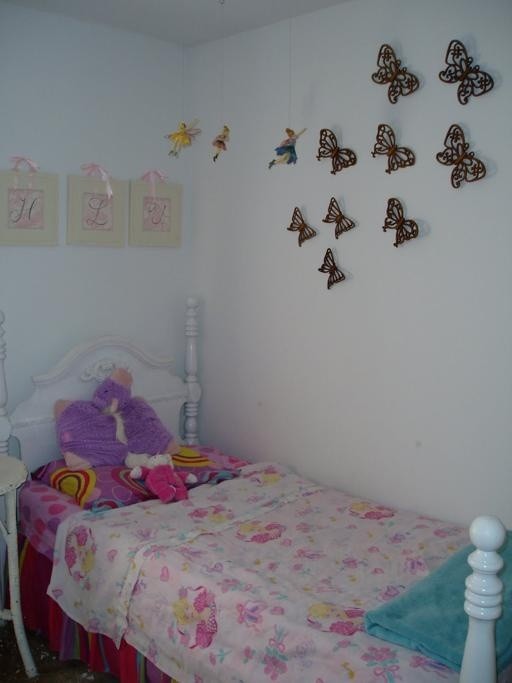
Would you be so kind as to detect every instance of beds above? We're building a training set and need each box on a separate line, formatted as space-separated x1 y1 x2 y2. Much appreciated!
0 295 511 683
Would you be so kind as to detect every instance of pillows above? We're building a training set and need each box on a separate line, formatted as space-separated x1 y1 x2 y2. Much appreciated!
31 447 238 513
55 368 184 471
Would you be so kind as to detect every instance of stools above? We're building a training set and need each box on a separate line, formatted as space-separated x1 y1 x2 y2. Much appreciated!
0 455 38 679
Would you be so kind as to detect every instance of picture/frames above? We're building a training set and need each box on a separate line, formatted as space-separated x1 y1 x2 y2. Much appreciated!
0 171 60 247
128 181 183 249
66 175 125 249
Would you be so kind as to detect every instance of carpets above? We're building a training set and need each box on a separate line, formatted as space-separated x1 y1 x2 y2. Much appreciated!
0 619 117 683
